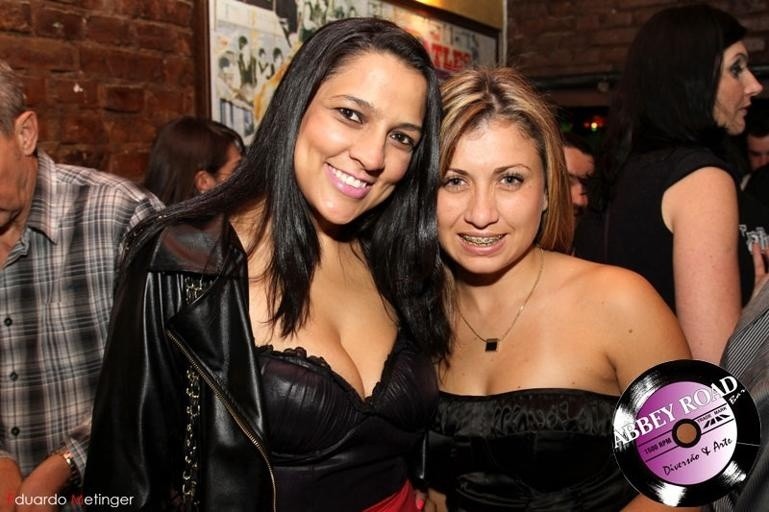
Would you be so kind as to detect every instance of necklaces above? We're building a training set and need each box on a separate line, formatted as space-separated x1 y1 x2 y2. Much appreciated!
452 246 544 352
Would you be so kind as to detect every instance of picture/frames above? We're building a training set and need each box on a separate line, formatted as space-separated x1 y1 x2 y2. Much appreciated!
195 0 502 147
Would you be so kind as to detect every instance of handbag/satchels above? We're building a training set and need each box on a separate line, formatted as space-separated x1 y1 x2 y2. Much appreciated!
143 273 199 511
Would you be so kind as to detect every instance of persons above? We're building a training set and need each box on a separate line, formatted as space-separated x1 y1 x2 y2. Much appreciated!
141 114 249 208
214 34 284 103
74 16 447 512
430 60 698 512
577 3 769 365
559 134 597 220
295 1 358 43
1 57 170 511
745 97 769 170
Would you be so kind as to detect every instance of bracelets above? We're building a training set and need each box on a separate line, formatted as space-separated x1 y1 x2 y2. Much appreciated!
48 444 82 488
